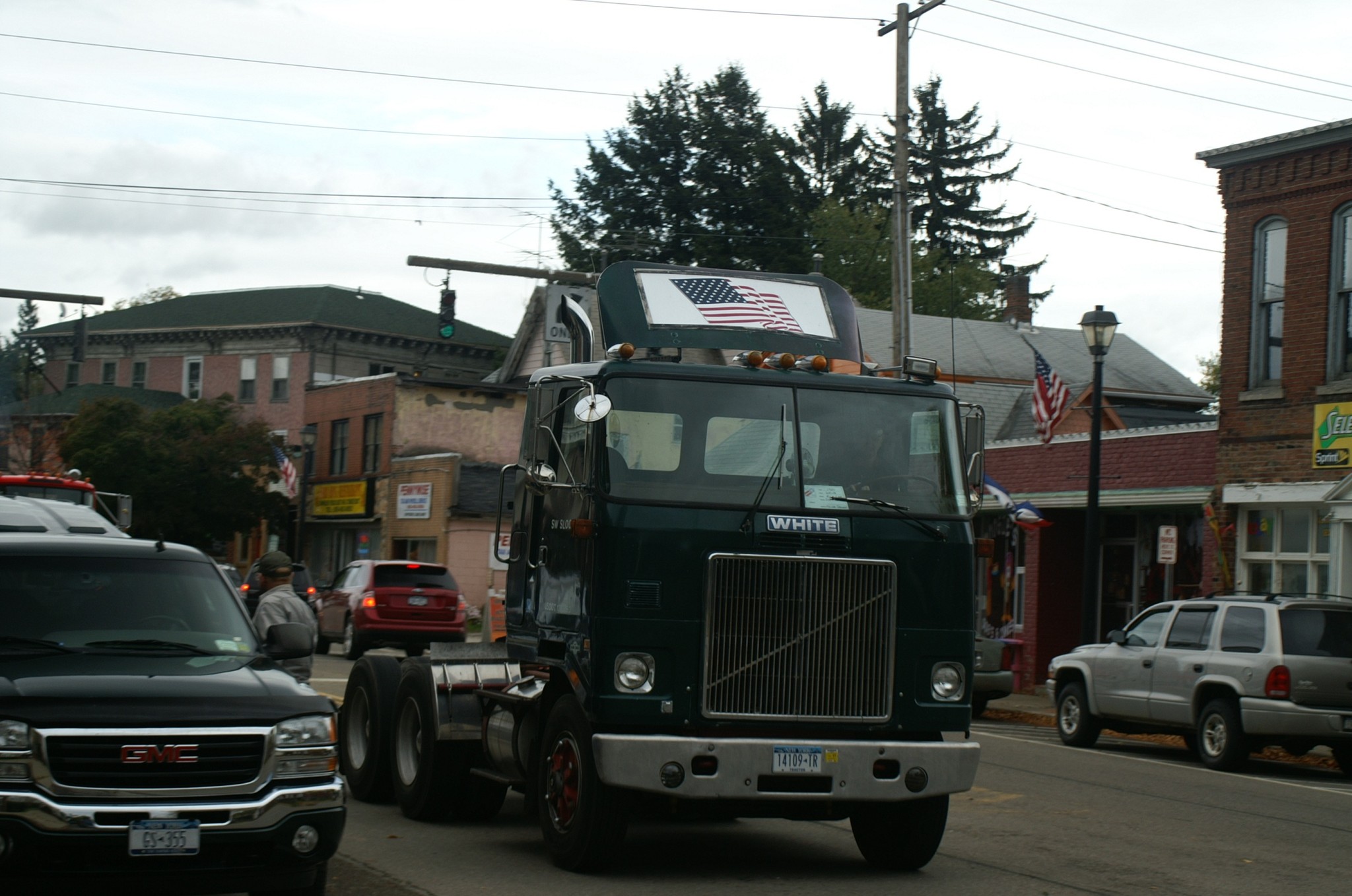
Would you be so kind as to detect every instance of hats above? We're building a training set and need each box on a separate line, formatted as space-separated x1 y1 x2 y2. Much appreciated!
257 550 293 579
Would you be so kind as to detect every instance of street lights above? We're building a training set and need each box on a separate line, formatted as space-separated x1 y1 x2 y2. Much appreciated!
293 424 319 564
1076 305 1123 637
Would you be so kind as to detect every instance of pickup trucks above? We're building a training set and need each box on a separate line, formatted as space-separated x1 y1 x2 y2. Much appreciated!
0 494 346 896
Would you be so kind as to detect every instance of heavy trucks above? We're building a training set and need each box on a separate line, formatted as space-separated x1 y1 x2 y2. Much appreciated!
338 265 1007 873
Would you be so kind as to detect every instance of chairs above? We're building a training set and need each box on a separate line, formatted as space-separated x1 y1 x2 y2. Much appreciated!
566 441 632 482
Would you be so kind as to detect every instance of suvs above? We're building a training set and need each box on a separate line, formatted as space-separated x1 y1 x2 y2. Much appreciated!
1046 590 1351 773
212 559 465 662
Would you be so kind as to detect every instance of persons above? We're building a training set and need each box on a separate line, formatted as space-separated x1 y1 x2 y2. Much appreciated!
243 551 315 684
816 419 904 490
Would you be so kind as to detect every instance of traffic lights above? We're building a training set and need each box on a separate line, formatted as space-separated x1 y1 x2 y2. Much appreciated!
439 289 456 336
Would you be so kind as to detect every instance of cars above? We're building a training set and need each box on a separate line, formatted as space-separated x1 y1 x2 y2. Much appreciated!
970 635 1014 718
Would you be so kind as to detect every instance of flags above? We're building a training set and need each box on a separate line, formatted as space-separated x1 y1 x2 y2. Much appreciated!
1031 348 1070 444
267 442 302 499
